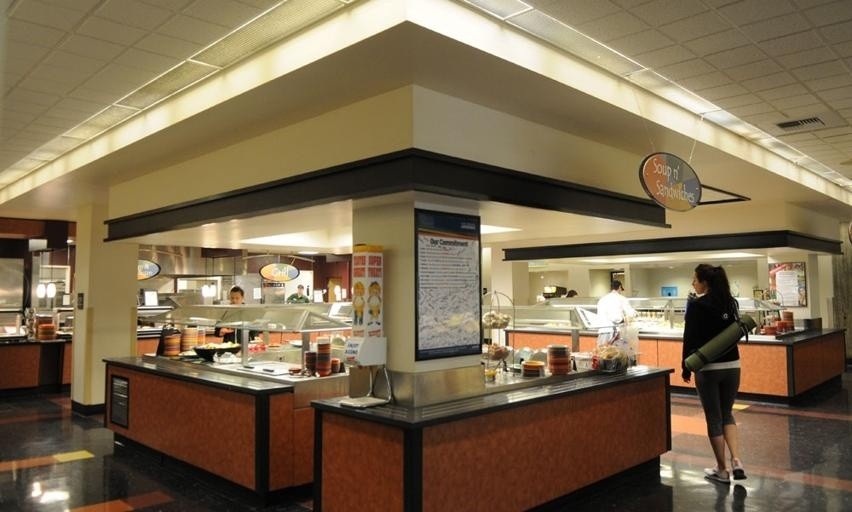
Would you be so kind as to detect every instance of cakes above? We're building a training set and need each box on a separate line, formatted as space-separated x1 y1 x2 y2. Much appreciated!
481 310 510 328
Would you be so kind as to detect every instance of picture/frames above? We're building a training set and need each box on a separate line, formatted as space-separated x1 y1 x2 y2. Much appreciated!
413 207 483 362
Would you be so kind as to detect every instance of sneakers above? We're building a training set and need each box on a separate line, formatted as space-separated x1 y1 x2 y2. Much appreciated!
731 457 747 480
703 465 730 482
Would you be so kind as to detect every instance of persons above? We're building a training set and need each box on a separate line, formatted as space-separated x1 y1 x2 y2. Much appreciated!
287 285 310 303
682 264 747 483
597 280 639 346
215 286 259 343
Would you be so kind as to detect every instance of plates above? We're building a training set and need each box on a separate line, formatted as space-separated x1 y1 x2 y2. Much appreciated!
763 310 793 335
522 361 544 378
38 324 55 340
164 327 198 356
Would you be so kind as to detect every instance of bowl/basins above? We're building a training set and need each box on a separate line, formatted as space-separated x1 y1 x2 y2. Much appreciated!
548 345 571 375
304 343 340 376
192 344 242 361
288 368 301 374
